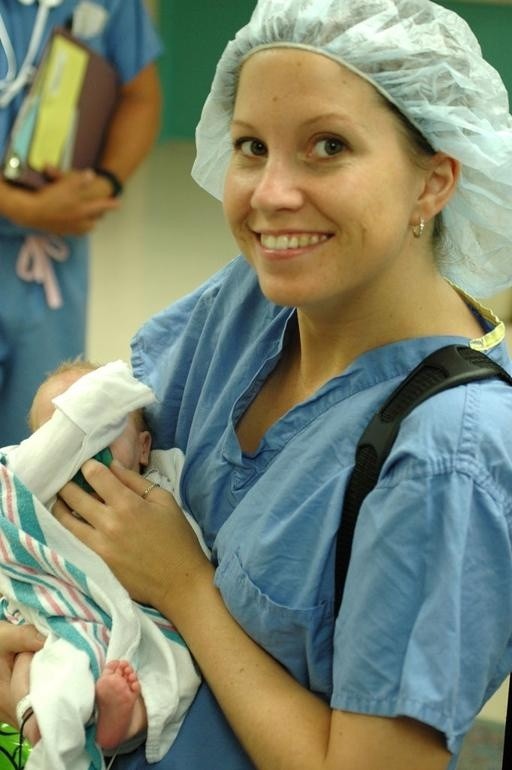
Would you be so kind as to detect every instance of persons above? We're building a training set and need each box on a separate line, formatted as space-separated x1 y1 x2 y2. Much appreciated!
10 352 148 749
0 0 512 770
1 0 166 448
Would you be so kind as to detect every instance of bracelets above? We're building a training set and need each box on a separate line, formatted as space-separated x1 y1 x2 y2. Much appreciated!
95 164 123 198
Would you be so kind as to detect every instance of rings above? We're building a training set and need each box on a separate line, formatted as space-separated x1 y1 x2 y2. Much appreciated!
141 483 160 498
